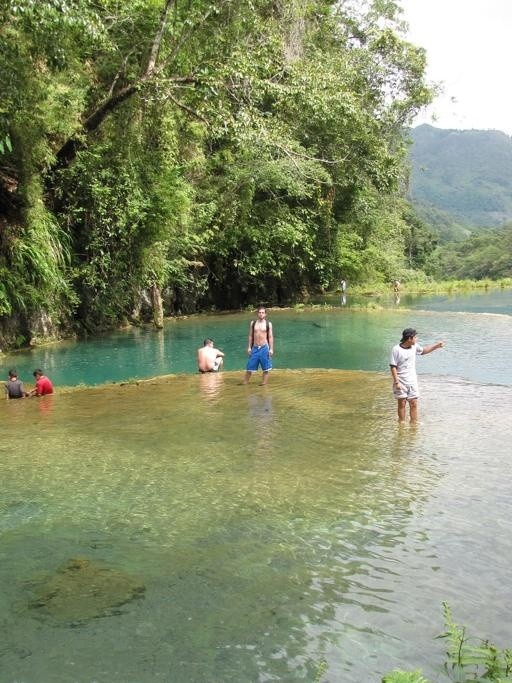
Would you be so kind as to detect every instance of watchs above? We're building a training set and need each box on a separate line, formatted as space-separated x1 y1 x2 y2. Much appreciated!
395 381 399 384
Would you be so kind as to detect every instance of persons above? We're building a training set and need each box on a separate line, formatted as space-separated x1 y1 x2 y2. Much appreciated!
341 294 347 306
389 327 446 422
243 306 274 384
394 292 400 305
197 338 225 372
340 278 346 293
4 369 25 400
26 368 54 398
393 280 401 292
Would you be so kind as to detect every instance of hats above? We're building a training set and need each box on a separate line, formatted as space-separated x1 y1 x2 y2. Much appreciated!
401 329 416 342
